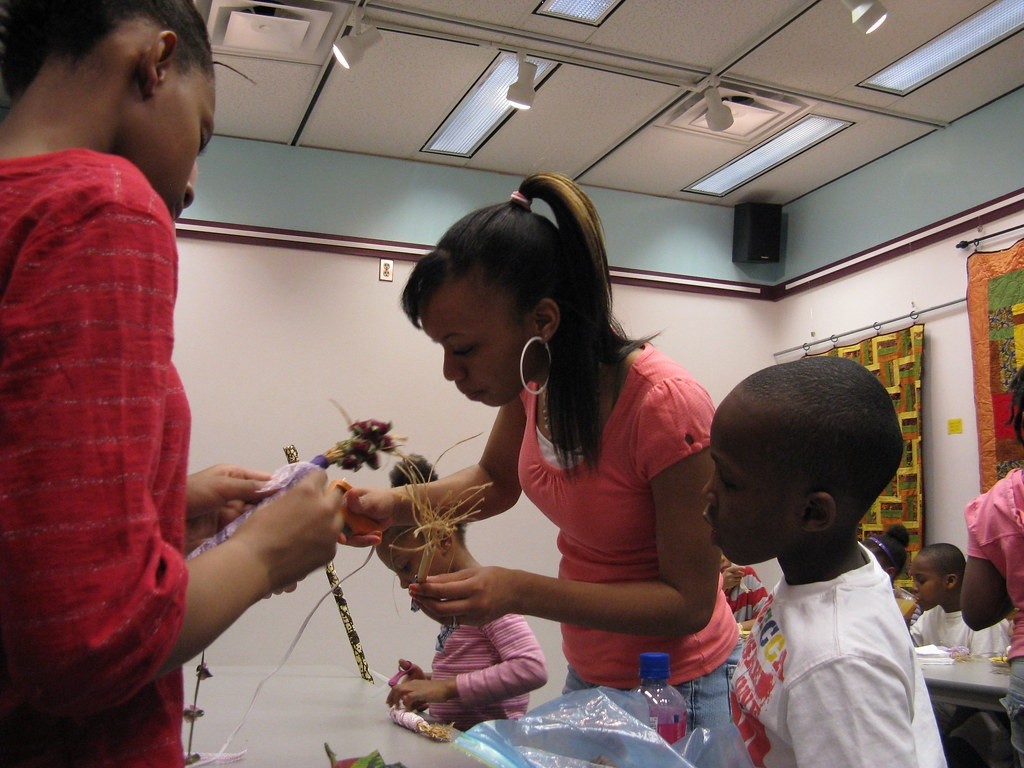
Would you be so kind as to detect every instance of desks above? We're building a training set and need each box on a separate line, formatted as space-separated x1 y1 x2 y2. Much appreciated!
182 660 494 767
919 656 1011 712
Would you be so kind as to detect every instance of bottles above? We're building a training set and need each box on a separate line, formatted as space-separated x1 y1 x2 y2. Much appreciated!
629 653 687 744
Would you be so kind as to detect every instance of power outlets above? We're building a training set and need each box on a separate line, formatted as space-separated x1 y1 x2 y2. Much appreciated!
379 259 394 282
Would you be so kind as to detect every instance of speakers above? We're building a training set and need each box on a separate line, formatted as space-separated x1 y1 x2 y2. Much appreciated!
732 202 781 265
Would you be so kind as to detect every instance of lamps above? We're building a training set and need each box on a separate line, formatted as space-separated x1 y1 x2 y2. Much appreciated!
507 49 537 110
703 87 733 133
841 0 888 34
331 7 382 71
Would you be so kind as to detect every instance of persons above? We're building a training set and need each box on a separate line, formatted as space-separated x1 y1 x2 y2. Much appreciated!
859 524 923 626
892 543 1010 658
960 364 1024 768
701 355 949 768
0 0 343 768
375 453 549 733
343 171 743 768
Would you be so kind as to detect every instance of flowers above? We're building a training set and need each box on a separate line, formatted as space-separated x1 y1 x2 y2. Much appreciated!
174 650 213 765
319 420 396 473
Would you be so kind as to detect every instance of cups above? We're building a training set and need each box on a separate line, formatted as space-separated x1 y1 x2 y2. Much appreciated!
892 587 919 622
